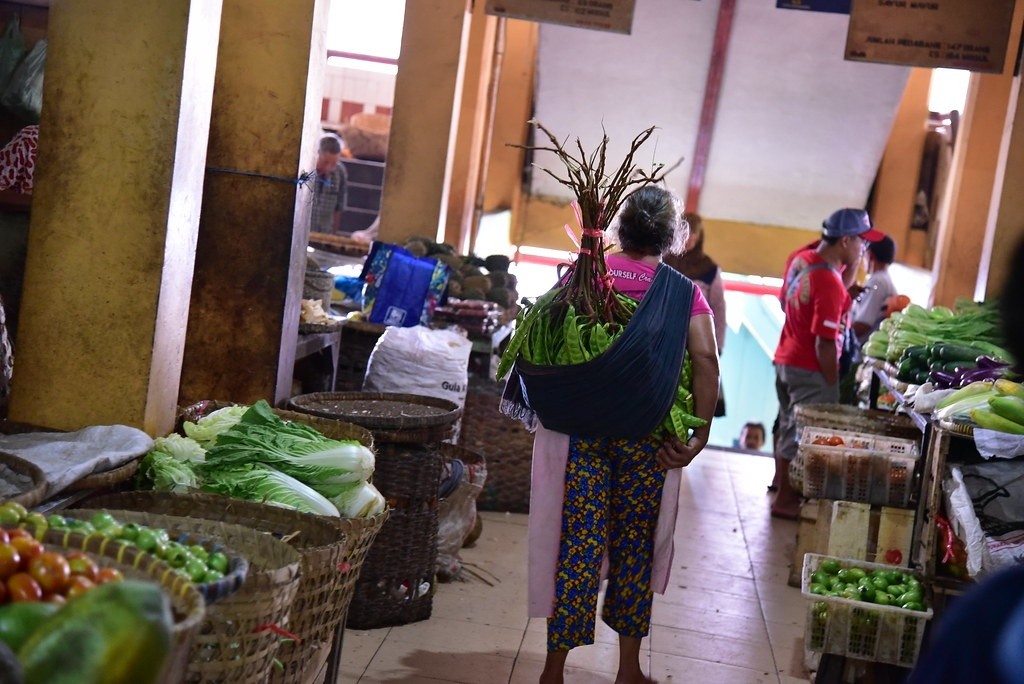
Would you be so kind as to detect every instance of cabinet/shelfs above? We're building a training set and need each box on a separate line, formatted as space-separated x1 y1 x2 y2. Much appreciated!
912 428 974 596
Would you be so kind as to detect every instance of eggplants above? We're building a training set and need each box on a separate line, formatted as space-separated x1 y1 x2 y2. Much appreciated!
928 355 1024 390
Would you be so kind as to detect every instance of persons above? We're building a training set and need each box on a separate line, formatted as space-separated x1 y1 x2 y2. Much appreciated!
309 135 348 232
739 207 897 520
537 186 721 684
661 210 727 356
0 124 41 434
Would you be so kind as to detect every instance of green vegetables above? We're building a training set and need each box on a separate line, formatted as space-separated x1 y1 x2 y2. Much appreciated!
860 297 1012 362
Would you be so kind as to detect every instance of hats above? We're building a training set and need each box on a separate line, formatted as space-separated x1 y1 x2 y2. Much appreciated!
820 208 885 242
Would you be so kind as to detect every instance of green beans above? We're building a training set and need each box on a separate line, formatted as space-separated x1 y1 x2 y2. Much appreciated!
496 290 709 446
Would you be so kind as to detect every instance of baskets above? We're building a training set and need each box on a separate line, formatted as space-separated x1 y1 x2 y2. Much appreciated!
788 403 975 673
13 384 534 684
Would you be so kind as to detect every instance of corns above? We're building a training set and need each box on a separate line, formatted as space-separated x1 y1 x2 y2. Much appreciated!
936 377 1024 436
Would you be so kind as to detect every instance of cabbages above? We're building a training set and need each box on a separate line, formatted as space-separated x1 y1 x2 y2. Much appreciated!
133 398 386 518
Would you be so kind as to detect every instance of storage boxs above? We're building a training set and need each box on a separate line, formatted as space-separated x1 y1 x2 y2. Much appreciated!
827 501 916 567
800 552 933 667
793 401 919 444
799 425 920 507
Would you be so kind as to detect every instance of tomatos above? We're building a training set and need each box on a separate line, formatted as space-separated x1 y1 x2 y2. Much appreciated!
0 499 228 606
807 557 924 663
805 437 909 508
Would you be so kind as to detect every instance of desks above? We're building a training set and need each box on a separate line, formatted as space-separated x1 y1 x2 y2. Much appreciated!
869 367 938 510
341 306 517 382
293 330 341 392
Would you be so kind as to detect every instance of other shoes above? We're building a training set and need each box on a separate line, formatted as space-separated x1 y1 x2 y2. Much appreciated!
770 506 801 522
